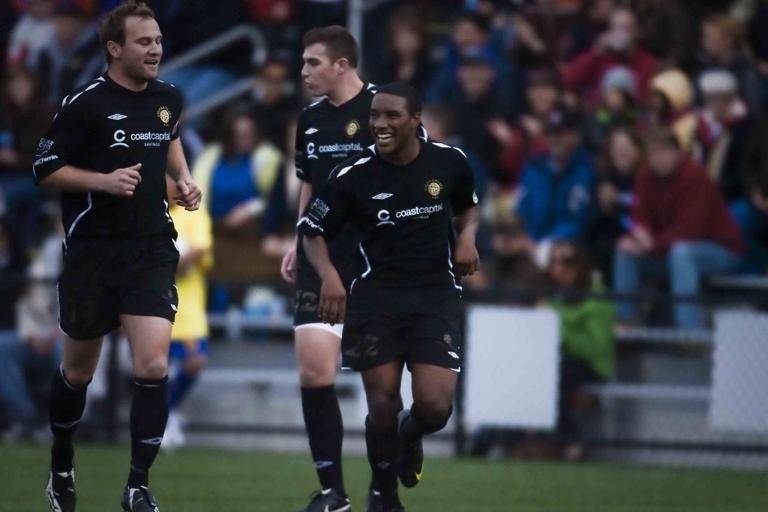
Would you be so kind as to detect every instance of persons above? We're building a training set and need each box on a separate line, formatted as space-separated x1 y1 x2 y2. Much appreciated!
27 1 204 512
279 24 427 512
296 82 482 512
1 1 768 455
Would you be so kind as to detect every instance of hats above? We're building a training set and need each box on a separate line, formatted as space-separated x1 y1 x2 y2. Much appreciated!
652 69 694 113
601 68 635 99
701 70 737 95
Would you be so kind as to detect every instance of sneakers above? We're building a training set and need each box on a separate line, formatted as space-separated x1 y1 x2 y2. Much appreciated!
120 481 161 512
396 409 425 488
367 479 407 511
302 487 353 512
46 461 77 511
